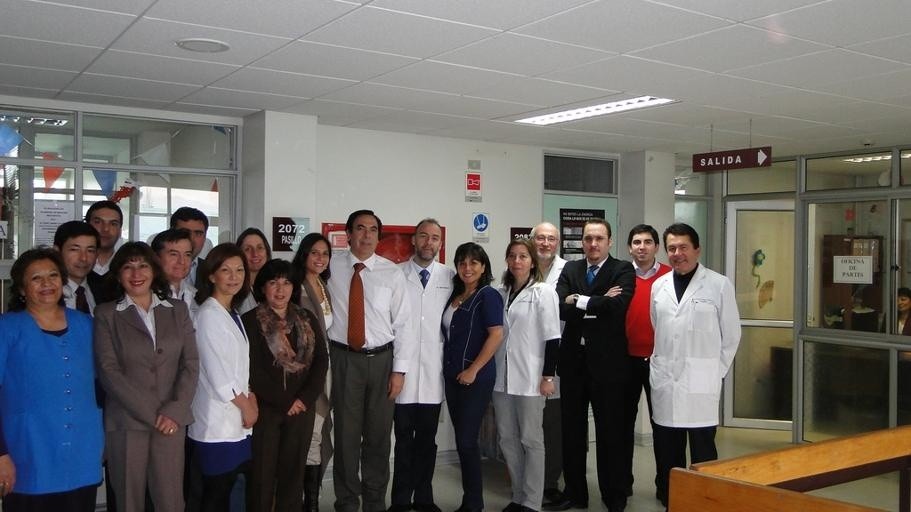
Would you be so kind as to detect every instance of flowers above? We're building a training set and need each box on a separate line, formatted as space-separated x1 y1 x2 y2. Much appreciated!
751 247 768 288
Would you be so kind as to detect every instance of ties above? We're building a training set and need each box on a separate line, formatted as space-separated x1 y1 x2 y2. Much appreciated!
586 265 599 284
420 270 429 288
75 286 89 313
348 263 365 352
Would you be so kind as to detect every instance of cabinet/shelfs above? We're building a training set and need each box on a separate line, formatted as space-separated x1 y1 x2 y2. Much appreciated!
821 235 887 332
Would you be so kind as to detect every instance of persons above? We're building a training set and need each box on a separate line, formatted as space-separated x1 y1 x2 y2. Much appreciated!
625 223 741 511
1 199 505 512
491 216 637 512
877 286 911 363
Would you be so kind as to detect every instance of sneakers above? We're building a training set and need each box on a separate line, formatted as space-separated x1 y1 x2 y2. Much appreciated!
388 503 442 512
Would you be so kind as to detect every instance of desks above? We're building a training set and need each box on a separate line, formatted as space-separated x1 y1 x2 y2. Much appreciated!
771 338 911 420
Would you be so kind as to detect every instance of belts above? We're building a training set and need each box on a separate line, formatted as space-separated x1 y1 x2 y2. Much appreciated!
331 340 392 356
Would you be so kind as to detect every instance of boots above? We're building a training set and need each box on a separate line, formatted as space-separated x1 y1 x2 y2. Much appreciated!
304 464 321 512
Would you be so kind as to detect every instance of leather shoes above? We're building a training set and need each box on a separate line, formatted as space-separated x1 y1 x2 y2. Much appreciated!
545 480 562 500
542 494 588 512
